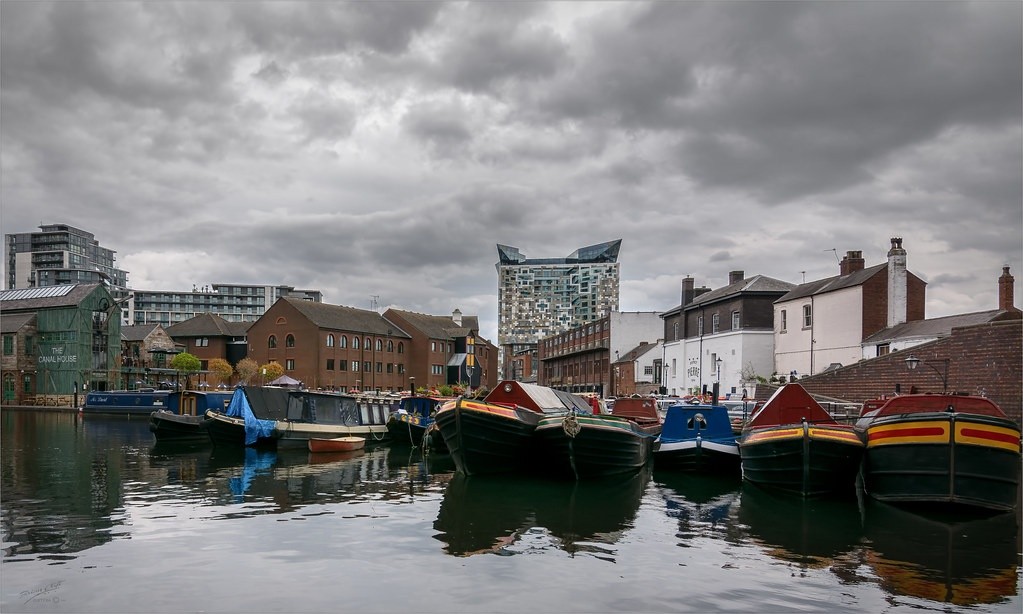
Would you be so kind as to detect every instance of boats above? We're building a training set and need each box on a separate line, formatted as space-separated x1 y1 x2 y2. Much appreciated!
308 436 366 453
147 389 236 445
78 376 174 415
420 379 1022 529
384 393 456 448
202 383 393 452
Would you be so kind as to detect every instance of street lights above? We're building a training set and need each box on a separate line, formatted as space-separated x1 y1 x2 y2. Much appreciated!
664 363 670 388
905 352 951 393
715 357 723 382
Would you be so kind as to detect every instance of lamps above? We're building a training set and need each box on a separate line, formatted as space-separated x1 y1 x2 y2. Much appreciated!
905 353 948 390
